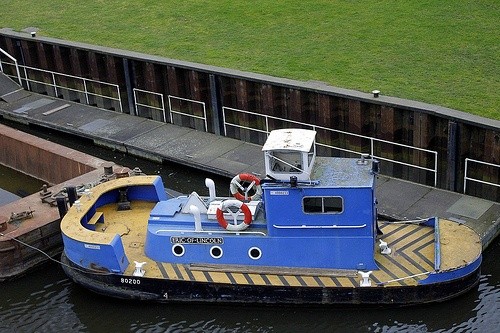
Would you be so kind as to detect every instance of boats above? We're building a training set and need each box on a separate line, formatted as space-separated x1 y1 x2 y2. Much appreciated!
59 127 482 306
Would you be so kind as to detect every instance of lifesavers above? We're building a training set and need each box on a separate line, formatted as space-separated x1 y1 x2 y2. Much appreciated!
230 174 263 201
216 200 252 231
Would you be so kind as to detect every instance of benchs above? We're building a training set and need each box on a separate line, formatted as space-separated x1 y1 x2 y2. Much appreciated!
81 204 104 231
